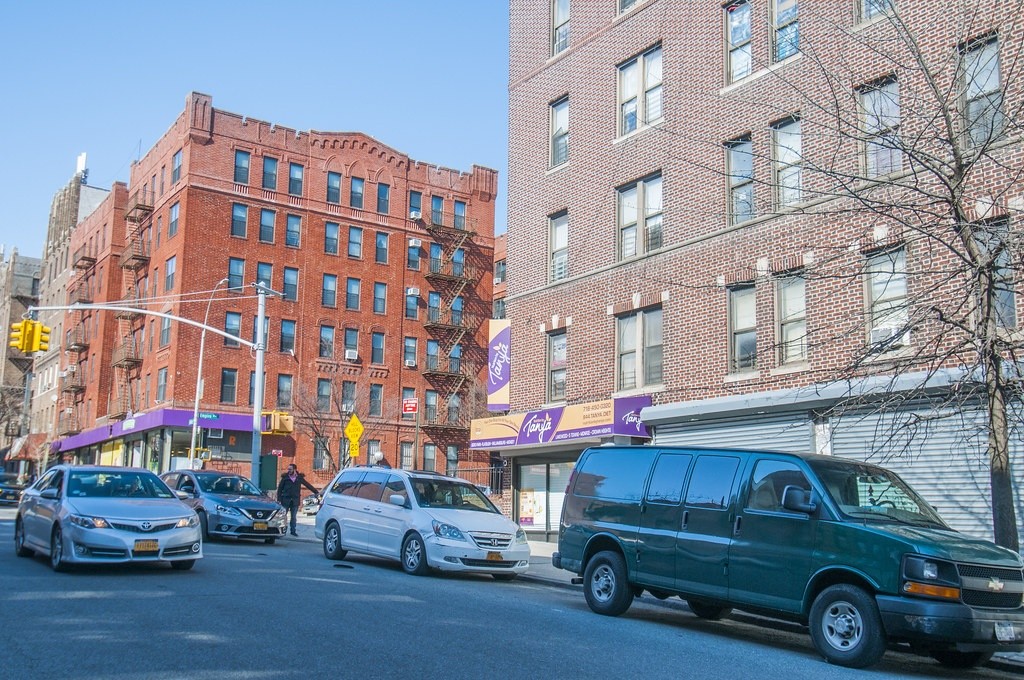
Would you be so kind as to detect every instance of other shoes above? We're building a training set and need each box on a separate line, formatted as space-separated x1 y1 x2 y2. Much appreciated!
290 531 298 536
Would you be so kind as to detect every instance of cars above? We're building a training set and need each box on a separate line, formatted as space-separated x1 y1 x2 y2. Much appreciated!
144 468 287 545
315 464 531 581
14 464 204 572
0 472 28 507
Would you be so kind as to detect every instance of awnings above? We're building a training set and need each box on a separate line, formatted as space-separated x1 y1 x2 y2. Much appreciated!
4 433 48 462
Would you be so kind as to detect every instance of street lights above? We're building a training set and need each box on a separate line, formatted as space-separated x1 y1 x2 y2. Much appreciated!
189 278 237 471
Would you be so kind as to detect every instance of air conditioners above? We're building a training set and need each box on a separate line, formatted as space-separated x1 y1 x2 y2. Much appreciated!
342 404 353 412
409 239 421 248
404 360 416 367
410 211 422 219
493 278 501 284
207 429 224 439
408 288 419 296
345 350 358 360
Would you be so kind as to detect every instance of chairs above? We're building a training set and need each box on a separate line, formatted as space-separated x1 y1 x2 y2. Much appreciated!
746 478 781 509
827 482 844 507
69 478 84 498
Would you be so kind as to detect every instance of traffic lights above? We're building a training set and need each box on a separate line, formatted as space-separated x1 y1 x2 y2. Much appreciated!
266 414 281 432
188 449 200 459
9 318 28 351
31 321 51 352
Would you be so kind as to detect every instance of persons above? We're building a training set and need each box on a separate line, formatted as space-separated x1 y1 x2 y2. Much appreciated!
277 464 321 537
128 477 141 495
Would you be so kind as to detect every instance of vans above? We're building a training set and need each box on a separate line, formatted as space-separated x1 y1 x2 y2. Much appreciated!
552 446 1024 670
